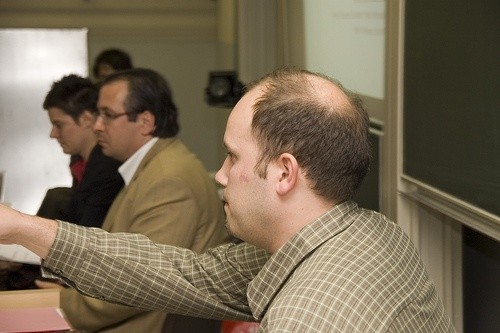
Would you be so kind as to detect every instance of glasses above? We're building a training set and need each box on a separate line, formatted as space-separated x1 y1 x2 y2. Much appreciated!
94 108 140 120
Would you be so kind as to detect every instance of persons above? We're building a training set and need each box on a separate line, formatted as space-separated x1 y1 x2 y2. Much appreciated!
0 64 458 333
0 46 232 333
